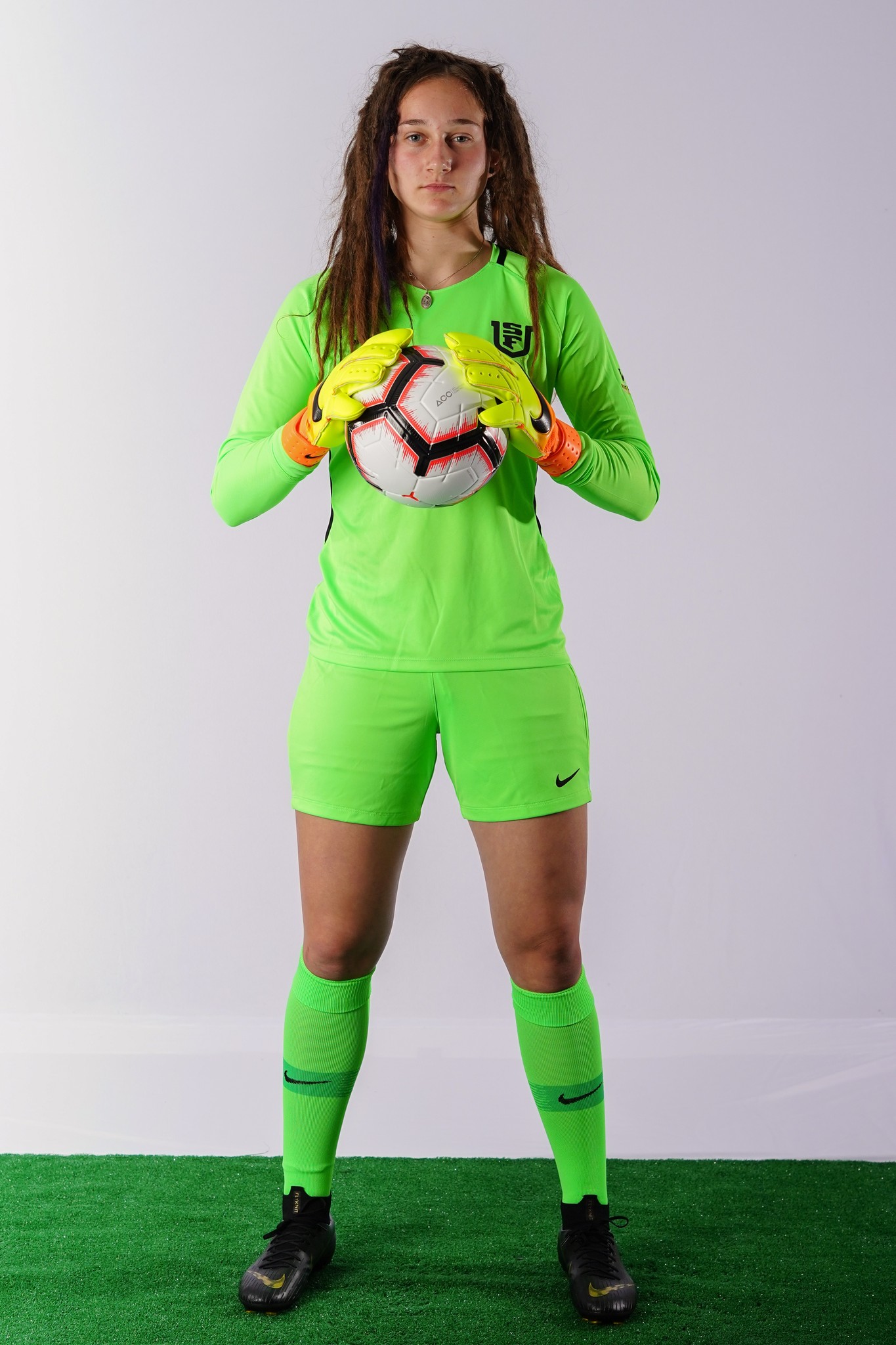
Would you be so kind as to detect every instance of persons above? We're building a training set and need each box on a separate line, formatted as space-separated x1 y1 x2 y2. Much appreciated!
208 47 660 1327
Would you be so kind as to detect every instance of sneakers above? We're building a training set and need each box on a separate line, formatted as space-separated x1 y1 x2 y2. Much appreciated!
239 1212 336 1315
557 1213 636 1325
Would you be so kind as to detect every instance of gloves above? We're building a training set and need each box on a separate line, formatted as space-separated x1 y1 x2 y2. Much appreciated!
443 331 560 463
299 329 415 447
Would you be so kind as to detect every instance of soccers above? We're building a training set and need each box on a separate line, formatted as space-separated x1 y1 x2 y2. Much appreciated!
344 344 511 510
